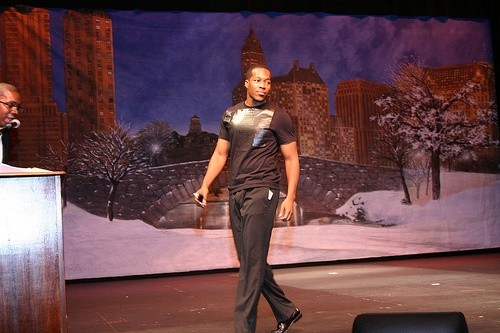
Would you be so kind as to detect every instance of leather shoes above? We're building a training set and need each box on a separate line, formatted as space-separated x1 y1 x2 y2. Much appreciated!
271 307 303 333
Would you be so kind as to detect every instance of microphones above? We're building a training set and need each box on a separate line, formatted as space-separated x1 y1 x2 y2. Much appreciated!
6 119 21 128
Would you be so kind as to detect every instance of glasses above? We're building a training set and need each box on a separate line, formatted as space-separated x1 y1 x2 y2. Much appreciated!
0 101 24 112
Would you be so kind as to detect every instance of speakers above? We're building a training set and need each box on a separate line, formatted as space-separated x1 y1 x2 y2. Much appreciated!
352 312 470 333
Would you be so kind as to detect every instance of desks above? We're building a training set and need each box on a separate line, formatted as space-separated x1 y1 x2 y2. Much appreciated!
0 171 67 333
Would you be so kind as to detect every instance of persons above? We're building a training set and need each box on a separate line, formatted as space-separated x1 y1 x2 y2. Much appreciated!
0 83 21 163
194 66 302 333
